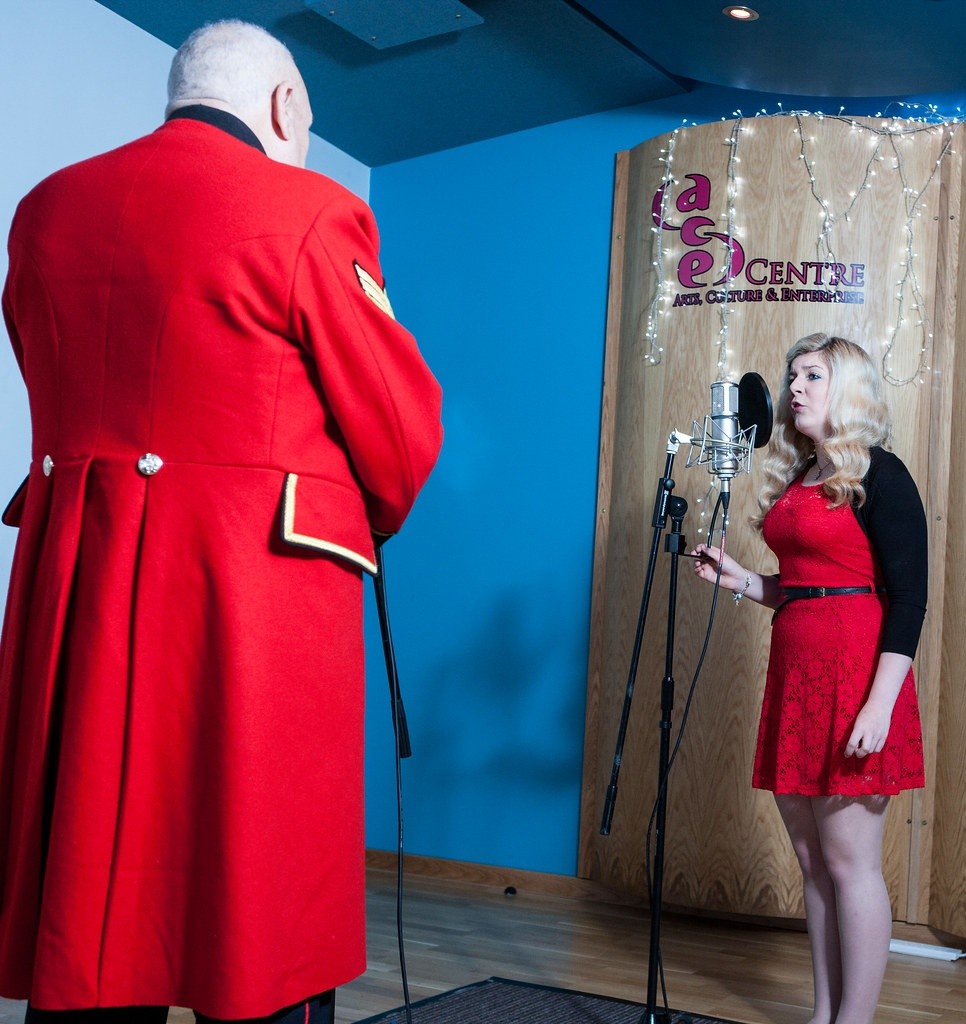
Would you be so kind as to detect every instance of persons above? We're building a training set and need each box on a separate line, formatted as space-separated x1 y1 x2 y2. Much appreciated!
1 17 444 1024
692 332 930 1024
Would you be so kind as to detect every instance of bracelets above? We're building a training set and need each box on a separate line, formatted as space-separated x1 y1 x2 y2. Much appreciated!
732 568 751 606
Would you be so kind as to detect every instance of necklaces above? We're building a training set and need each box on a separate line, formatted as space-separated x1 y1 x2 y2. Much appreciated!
814 462 831 479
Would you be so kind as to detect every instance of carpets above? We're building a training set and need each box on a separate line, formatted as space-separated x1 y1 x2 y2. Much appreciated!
350 976 752 1024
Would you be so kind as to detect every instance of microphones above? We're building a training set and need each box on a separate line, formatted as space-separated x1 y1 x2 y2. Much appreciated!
711 381 740 509
